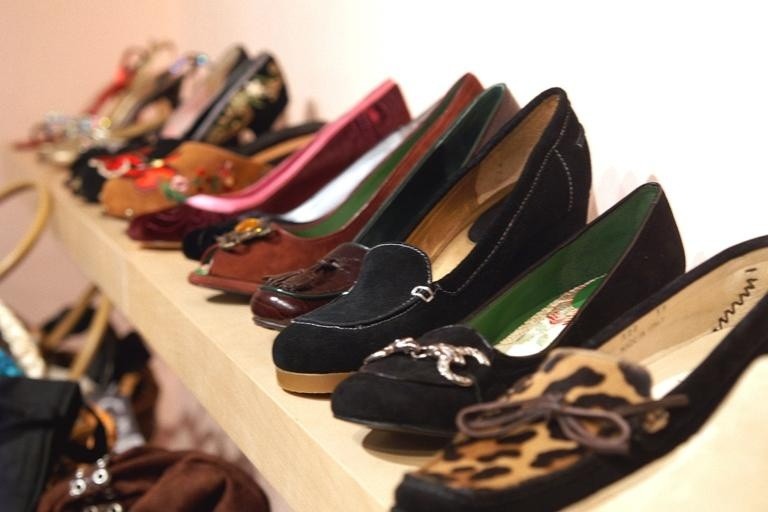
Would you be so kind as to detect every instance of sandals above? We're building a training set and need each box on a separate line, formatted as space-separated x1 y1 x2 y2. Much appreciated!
12 41 768 512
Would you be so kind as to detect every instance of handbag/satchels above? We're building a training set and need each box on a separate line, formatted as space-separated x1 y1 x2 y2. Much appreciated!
0 283 273 511
